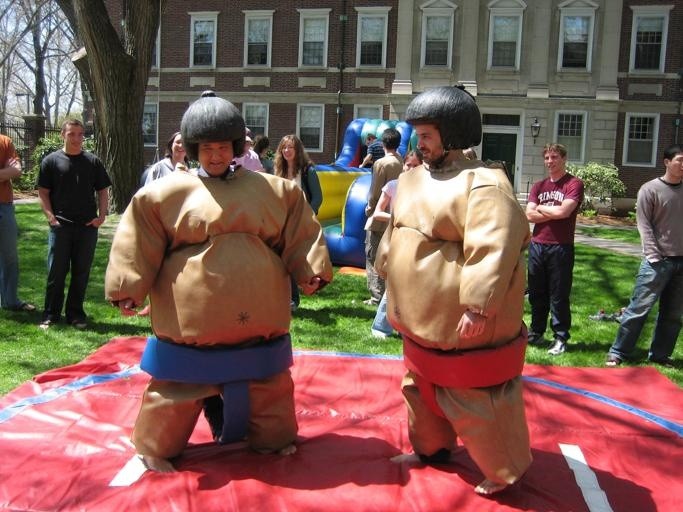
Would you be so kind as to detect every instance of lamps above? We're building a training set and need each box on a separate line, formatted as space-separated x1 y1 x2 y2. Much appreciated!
530 115 539 145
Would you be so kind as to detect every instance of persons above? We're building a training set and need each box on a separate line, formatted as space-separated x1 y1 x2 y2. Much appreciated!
373 87 532 494
39 118 112 329
524 144 585 354
145 130 191 187
363 128 404 304
232 127 274 176
104 91 332 474
371 149 424 340
1 133 35 311
274 134 323 311
607 146 683 368
358 133 384 169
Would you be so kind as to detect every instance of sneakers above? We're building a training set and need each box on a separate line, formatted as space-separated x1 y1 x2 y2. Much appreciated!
362 296 381 306
2 301 37 313
371 328 388 341
66 315 88 330
290 299 300 312
605 353 620 367
547 337 566 356
650 357 673 368
138 304 151 316
39 317 58 330
526 328 544 345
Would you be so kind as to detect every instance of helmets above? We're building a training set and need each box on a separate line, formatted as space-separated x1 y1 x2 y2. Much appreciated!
179 90 245 161
404 84 482 151
